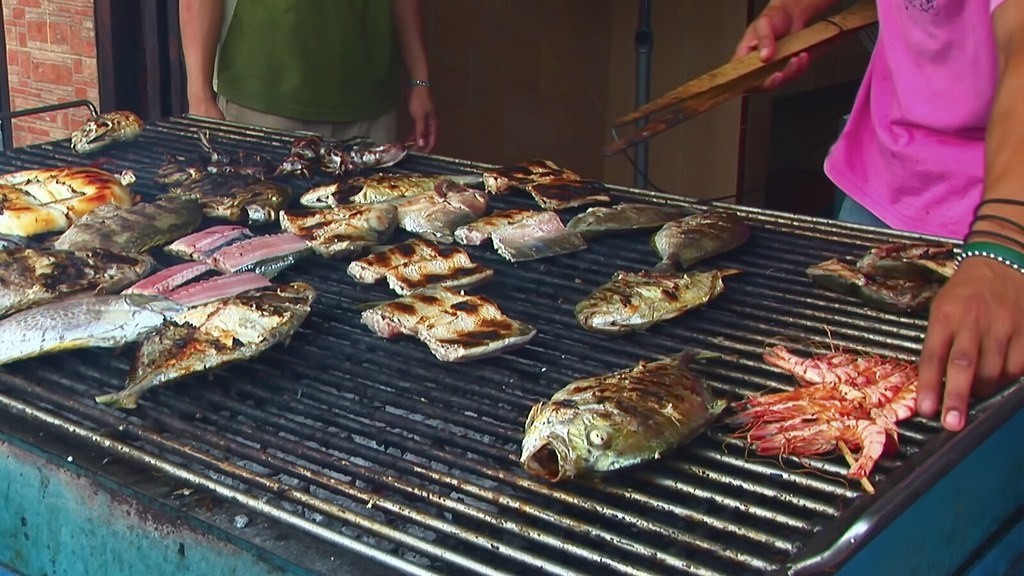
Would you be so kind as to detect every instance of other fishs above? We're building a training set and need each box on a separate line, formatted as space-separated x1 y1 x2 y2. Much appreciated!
1 109 962 484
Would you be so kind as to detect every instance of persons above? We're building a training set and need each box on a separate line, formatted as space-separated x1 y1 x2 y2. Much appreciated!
179 0 436 154
729 0 1023 432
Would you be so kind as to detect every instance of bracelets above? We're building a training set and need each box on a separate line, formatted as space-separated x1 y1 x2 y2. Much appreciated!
953 198 1024 273
406 80 431 87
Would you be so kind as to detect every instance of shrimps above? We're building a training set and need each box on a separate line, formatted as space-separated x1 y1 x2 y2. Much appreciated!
722 324 920 481
